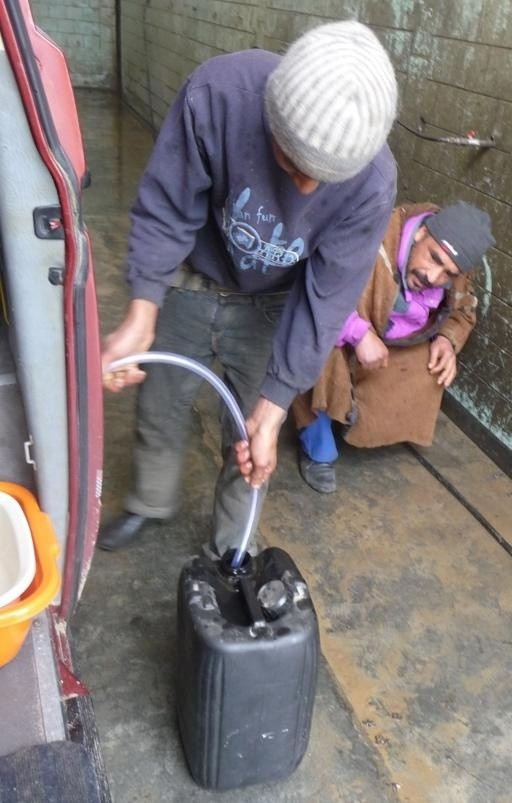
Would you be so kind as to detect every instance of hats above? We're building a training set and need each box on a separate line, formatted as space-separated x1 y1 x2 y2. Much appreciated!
423 199 496 273
264 20 398 184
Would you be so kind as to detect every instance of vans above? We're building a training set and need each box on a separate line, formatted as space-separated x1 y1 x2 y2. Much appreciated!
0 0 108 803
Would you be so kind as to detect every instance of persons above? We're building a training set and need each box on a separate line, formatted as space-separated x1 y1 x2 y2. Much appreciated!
287 196 499 494
92 17 405 566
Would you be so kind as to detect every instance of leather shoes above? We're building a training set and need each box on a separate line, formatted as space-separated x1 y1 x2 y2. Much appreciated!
96 510 162 551
299 445 337 493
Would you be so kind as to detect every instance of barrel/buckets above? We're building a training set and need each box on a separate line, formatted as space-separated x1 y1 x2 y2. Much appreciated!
172 547 320 792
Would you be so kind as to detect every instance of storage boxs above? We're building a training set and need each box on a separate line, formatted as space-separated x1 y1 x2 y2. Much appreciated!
0 481 62 668
